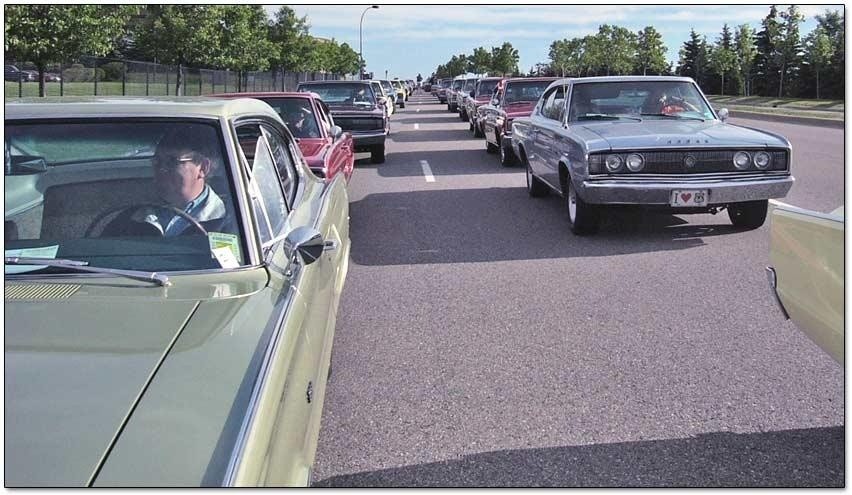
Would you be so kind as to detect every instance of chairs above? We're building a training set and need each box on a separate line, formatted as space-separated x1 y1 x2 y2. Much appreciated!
509 86 528 101
572 94 599 114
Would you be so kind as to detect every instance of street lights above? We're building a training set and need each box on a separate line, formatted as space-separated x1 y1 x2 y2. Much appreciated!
360 4 380 79
775 49 786 97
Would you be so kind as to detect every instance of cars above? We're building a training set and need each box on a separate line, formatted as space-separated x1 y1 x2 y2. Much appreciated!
286 80 389 163
5 97 352 488
348 74 516 138
6 64 61 82
764 189 844 372
511 75 795 234
477 77 601 165
195 90 355 192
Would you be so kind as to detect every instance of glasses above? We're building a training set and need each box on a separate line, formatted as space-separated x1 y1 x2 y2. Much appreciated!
152 154 192 171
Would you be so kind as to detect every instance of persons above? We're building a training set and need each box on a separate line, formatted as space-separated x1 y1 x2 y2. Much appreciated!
642 89 683 114
344 86 371 107
414 71 422 95
95 127 238 240
287 109 319 137
